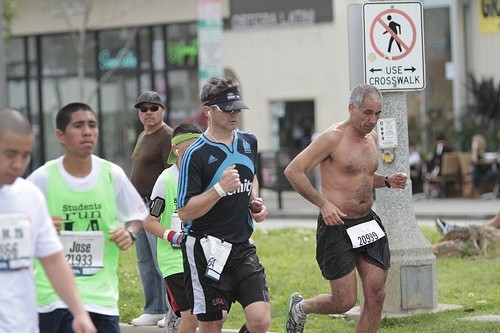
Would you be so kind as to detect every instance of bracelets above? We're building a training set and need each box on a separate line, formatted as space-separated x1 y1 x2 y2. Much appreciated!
162 229 183 248
214 182 227 198
384 175 390 189
249 197 264 208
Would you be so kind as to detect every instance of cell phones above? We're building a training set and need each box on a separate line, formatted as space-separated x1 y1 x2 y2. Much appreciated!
151 197 165 217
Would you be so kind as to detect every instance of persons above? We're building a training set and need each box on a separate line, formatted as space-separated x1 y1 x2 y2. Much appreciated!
177 78 271 333
0 108 98 333
26 103 149 333
463 133 490 197
285 85 408 333
435 184 500 235
127 91 174 328
408 141 421 180
141 121 199 331
494 129 500 200
422 131 454 195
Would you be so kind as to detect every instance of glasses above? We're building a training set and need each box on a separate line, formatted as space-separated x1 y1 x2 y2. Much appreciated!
138 106 159 112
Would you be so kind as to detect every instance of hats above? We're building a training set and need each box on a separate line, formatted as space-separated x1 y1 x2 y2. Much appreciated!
135 91 164 109
202 86 250 111
167 133 202 164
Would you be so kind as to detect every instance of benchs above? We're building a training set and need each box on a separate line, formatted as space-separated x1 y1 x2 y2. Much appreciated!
425 153 463 196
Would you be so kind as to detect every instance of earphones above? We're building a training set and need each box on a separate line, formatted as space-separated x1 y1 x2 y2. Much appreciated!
176 149 180 155
207 111 212 117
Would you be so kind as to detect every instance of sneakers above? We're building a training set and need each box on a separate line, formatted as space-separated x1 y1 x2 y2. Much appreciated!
157 315 166 327
284 292 306 333
165 307 182 333
132 313 165 325
435 217 457 236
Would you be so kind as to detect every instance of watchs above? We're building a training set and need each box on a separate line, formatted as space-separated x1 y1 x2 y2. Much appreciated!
126 229 138 241
